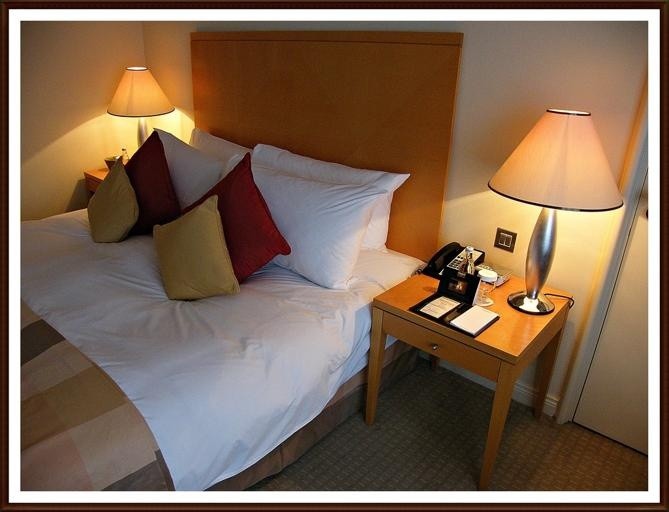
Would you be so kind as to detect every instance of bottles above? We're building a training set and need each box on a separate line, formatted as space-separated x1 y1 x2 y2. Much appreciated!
459 246 476 275
121 148 129 166
474 269 498 307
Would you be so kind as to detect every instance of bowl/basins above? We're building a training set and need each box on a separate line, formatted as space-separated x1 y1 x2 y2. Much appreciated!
105 156 131 172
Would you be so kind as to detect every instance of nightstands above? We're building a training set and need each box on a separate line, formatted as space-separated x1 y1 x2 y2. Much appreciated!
364 266 575 490
84 168 111 207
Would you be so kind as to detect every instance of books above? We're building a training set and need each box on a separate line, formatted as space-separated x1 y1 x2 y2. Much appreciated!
407 291 500 339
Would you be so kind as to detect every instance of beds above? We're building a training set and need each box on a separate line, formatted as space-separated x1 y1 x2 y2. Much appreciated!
20 23 465 490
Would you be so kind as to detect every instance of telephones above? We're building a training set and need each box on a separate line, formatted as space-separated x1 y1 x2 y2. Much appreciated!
422 242 485 281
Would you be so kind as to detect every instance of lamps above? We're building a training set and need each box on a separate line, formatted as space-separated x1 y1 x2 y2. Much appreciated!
486 109 624 316
106 66 176 151
